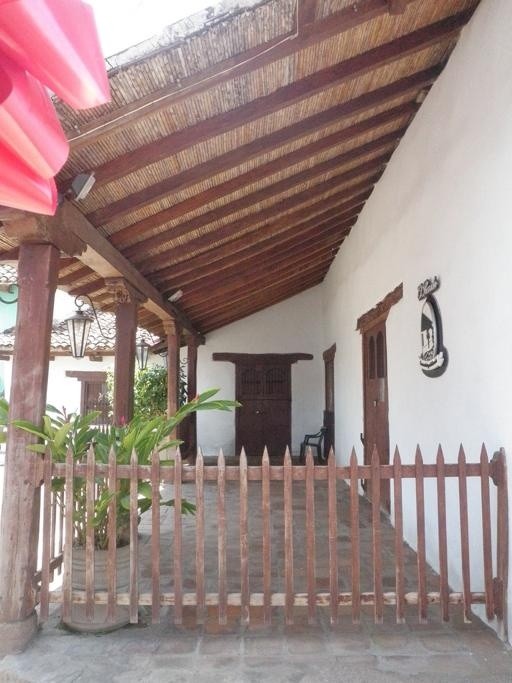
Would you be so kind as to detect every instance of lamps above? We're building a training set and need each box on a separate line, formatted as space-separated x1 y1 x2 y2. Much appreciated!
134 325 169 372
63 293 116 359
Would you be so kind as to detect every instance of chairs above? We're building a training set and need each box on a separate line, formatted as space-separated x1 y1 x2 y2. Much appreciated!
298 426 329 464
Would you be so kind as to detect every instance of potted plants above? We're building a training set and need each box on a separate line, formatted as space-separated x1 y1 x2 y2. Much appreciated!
133 364 189 463
12 386 243 636
